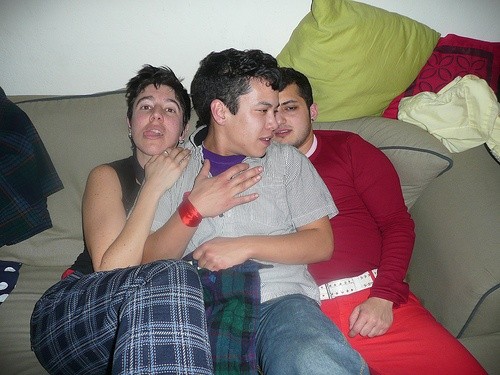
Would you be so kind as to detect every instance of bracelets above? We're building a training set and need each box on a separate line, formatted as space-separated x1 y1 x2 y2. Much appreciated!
177 192 205 228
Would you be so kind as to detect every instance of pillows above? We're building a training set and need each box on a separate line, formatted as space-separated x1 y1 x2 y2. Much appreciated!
275 0 441 123
382 34 500 121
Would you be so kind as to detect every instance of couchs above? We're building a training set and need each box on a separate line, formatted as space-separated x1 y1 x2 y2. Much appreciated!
1 87 500 375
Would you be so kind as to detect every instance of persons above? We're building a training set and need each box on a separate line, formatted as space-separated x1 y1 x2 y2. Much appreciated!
30 62 216 375
126 47 371 375
271 67 489 375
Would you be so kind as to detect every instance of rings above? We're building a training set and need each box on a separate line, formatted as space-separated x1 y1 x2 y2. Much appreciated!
163 149 169 155
